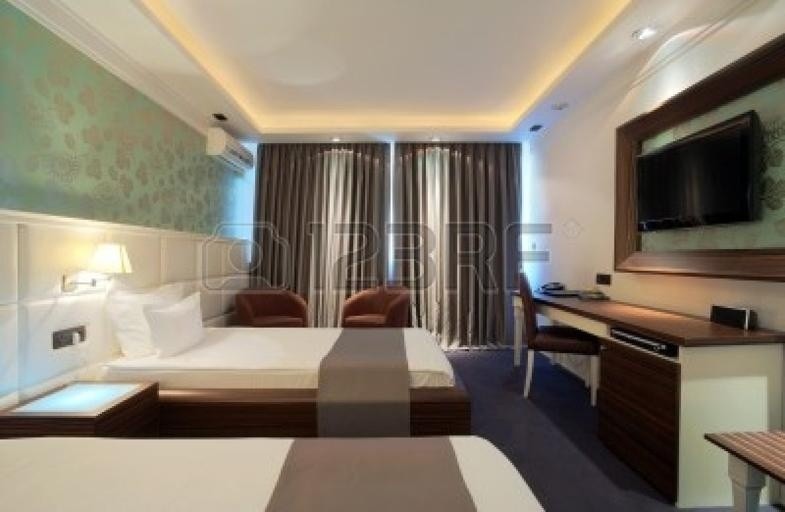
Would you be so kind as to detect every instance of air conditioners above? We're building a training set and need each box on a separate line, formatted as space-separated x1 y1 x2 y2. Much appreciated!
201 124 257 176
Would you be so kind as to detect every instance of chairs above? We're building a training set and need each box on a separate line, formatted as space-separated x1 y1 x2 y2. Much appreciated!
232 285 311 328
339 284 413 330
514 269 601 410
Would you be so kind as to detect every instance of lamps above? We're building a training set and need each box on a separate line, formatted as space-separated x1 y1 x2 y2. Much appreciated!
54 236 135 295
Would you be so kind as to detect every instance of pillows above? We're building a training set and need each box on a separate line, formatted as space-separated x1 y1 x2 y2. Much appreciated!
139 283 187 358
106 281 170 362
148 287 205 358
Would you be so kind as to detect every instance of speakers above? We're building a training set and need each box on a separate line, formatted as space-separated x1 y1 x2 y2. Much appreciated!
709 304 758 331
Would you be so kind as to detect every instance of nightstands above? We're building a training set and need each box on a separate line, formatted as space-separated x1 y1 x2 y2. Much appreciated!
1 376 164 440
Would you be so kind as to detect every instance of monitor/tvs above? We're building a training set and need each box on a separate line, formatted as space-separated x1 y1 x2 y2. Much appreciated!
636 109 761 232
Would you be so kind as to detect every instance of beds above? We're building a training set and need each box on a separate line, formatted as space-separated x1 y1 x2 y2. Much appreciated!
92 322 475 439
0 429 546 510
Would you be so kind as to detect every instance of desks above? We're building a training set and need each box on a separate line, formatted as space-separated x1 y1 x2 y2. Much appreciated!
699 426 785 508
509 284 785 510
